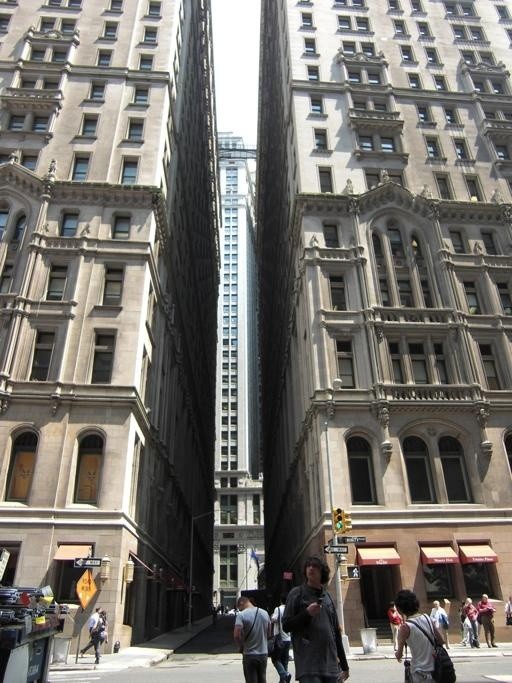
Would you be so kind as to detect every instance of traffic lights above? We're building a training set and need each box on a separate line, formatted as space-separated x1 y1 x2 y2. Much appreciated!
332 508 353 535
347 565 361 580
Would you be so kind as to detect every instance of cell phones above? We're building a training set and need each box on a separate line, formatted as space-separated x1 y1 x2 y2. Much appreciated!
317 598 323 606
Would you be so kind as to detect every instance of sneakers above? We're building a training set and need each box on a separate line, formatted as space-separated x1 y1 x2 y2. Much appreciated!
488 644 498 648
79 650 100 664
279 674 291 683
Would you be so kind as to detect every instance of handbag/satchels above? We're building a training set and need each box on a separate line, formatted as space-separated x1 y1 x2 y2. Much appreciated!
440 614 450 630
431 645 456 683
267 632 285 657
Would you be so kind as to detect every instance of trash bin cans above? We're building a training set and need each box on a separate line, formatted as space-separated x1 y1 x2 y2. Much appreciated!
360 628 377 654
52 636 72 664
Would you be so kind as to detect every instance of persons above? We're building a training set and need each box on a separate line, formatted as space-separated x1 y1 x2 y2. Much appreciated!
280 554 350 683
231 594 272 683
87 606 102 657
81 605 109 666
393 587 447 683
389 592 512 652
211 602 236 627
269 596 292 683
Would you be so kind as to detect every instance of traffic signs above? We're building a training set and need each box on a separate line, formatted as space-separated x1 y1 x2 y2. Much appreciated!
323 536 366 554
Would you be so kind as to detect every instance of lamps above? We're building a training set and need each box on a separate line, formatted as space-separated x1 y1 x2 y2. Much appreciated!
338 553 348 580
100 554 111 582
123 557 135 584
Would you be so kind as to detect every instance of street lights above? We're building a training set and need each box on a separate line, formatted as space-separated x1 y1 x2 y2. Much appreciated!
324 378 350 656
187 510 232 632
